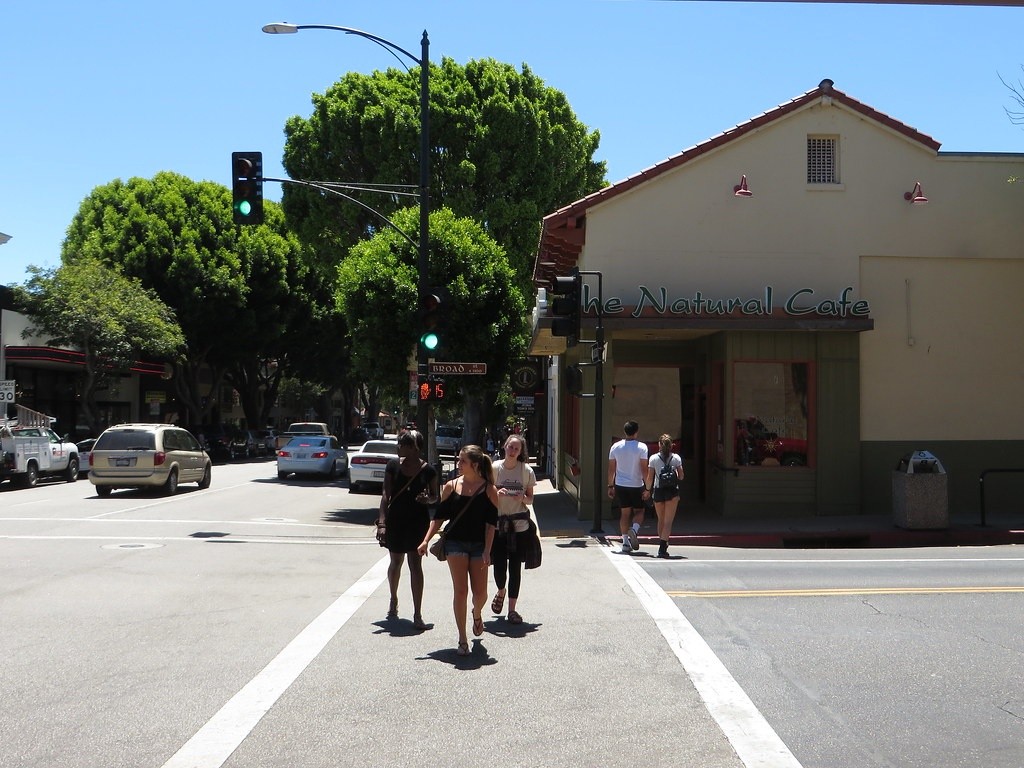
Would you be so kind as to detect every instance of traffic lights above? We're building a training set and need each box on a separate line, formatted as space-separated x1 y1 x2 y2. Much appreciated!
416 379 448 403
565 366 584 395
230 151 264 226
551 267 581 347
418 285 443 356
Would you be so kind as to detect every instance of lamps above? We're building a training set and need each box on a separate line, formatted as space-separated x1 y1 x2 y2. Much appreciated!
734 174 752 197
903 181 928 205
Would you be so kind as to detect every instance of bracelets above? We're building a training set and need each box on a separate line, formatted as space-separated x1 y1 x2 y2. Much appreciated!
607 485 614 488
646 489 649 491
378 524 387 527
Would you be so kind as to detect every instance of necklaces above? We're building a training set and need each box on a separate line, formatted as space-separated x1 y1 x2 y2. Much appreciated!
464 483 477 494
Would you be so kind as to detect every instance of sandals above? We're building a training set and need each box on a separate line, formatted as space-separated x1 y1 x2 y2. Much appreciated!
508 610 523 624
491 587 506 614
458 641 469 656
472 608 483 636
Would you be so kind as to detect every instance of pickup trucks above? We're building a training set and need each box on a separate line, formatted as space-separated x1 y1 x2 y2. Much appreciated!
275 421 333 450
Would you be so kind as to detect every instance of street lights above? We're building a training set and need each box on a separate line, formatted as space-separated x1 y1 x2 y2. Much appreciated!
260 22 434 461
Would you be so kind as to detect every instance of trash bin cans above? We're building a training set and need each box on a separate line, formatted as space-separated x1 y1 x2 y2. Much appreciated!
890 451 949 529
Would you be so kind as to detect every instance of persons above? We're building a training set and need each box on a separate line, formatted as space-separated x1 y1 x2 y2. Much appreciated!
736 421 750 466
607 419 649 552
642 434 684 557
748 415 767 465
376 430 441 630
491 434 541 624
417 444 498 657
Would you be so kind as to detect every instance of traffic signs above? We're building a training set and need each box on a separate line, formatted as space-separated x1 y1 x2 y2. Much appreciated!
590 341 603 365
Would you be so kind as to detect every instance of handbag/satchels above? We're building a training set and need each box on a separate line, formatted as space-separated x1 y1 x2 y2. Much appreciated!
375 500 390 548
429 536 448 562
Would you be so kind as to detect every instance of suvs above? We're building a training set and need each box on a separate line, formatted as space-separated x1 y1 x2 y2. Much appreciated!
87 423 212 496
0 418 81 490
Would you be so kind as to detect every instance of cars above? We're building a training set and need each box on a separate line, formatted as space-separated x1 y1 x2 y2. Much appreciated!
434 423 464 456
349 439 398 492
75 438 97 479
359 422 385 440
733 415 808 468
191 422 282 463
405 422 417 431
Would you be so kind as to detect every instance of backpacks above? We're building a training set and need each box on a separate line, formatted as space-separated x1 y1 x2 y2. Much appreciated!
654 454 677 489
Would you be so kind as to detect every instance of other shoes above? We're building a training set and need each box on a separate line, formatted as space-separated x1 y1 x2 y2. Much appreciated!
628 528 639 550
656 540 669 557
414 613 425 629
386 599 399 620
622 544 633 552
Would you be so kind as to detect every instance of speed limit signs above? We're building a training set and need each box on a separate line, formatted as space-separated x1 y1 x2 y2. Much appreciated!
0 380 16 404
